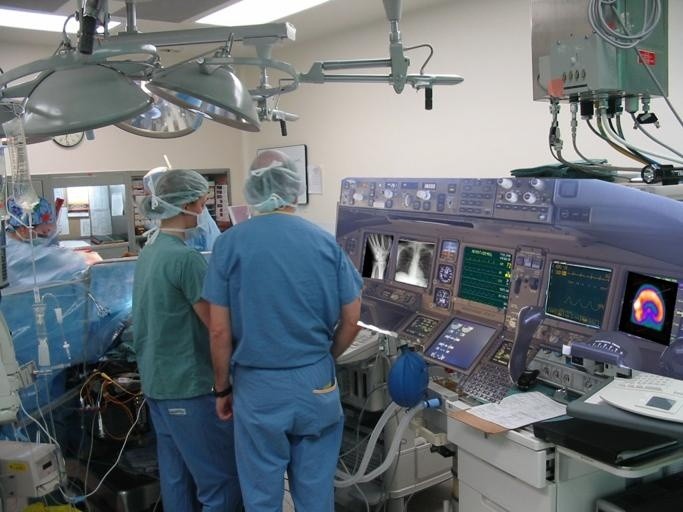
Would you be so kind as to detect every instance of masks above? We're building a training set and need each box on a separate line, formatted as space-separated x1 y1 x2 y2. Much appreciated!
184 207 212 240
21 231 49 248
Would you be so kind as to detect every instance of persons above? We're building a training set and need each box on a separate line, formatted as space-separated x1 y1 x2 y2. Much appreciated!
131 169 245 512
199 147 365 512
122 168 222 257
2 191 104 291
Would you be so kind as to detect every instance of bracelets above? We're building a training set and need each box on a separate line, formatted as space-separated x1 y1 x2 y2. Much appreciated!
211 384 232 397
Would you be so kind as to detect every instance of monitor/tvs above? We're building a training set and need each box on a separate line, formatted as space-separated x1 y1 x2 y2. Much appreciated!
358 295 413 331
417 315 502 374
544 257 620 330
459 245 516 315
356 232 395 282
613 268 682 350
391 234 438 295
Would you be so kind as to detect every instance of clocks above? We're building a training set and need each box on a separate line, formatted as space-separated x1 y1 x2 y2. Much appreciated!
50 131 84 148
